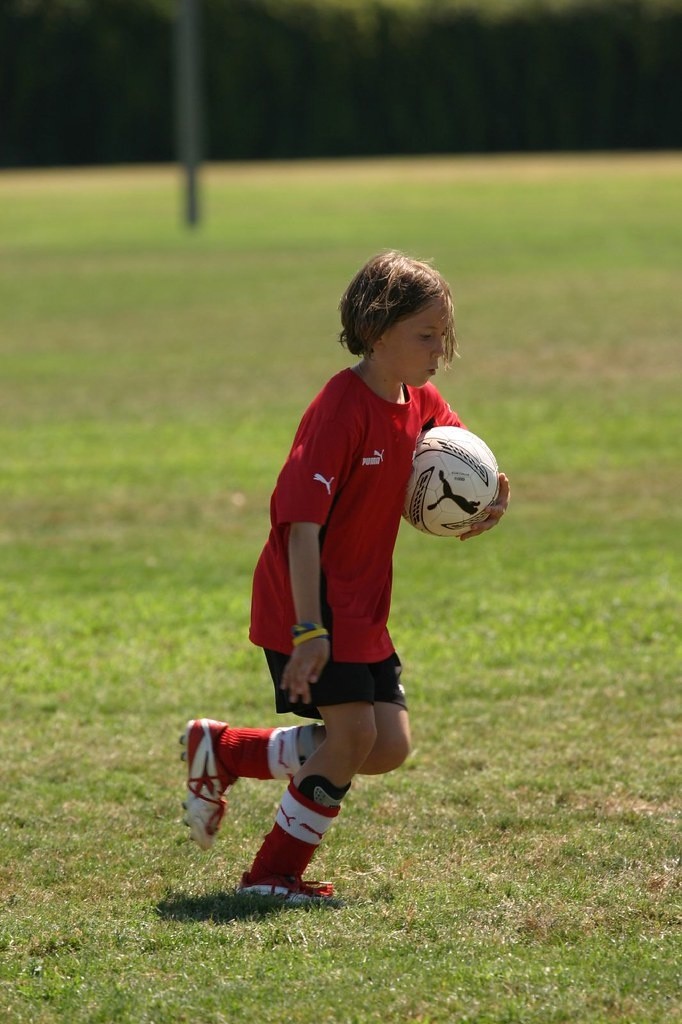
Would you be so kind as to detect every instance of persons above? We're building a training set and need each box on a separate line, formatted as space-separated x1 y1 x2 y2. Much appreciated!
175 251 510 904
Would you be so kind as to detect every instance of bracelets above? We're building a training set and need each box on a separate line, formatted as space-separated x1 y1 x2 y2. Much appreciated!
287 623 332 646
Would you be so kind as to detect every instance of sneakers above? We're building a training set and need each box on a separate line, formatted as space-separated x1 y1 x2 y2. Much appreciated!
179 718 239 851
235 872 346 904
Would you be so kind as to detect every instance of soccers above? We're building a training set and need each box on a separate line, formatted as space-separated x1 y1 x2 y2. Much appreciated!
398 427 499 535
